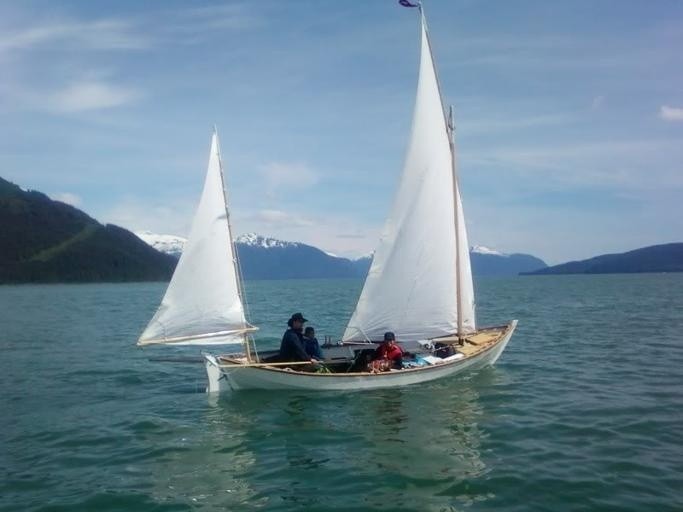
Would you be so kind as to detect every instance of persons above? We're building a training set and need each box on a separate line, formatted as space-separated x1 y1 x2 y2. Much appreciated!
303 327 324 361
280 313 331 373
371 332 404 371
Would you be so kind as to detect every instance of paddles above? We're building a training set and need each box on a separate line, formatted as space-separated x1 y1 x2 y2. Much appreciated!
205 355 324 367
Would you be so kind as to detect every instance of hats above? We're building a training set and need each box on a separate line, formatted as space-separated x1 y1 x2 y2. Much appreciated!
288 313 308 327
385 332 394 341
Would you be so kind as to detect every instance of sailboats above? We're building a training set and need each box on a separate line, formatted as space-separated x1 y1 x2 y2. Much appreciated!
136 0 519 396
